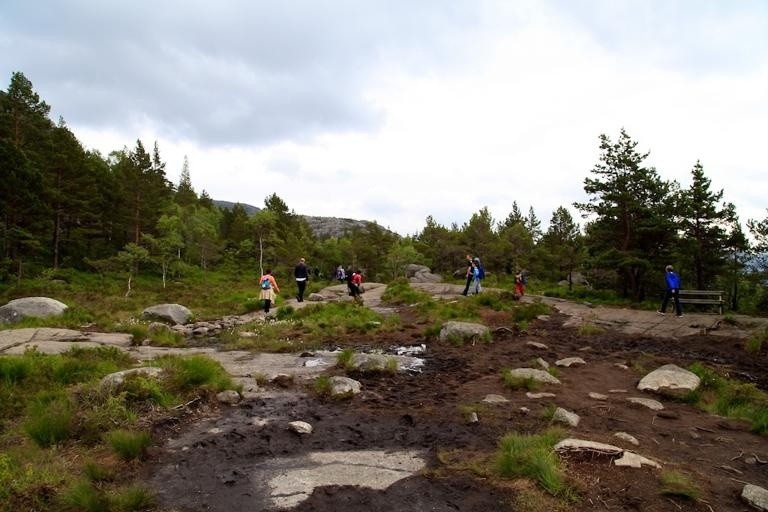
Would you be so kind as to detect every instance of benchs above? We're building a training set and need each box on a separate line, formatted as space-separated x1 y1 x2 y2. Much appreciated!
672 290 726 315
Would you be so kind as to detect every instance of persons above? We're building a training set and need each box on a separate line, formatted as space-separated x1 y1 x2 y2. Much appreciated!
294 258 309 302
314 263 361 297
257 268 280 313
461 252 527 298
658 265 683 318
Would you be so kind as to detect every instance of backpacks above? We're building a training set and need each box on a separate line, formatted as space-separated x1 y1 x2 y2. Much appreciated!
261 278 271 289
477 268 485 279
